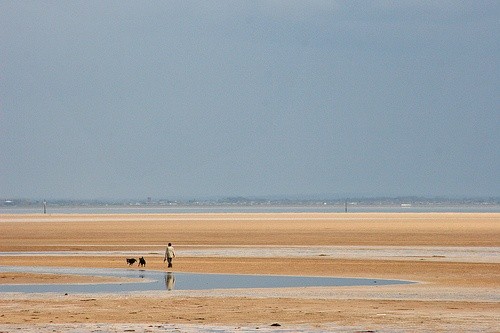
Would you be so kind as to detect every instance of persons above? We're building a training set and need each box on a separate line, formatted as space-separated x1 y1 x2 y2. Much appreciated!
163 243 177 267
163 272 176 291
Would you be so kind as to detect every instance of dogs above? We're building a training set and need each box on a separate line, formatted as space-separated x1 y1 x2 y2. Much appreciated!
126 258 137 266
138 256 146 267
